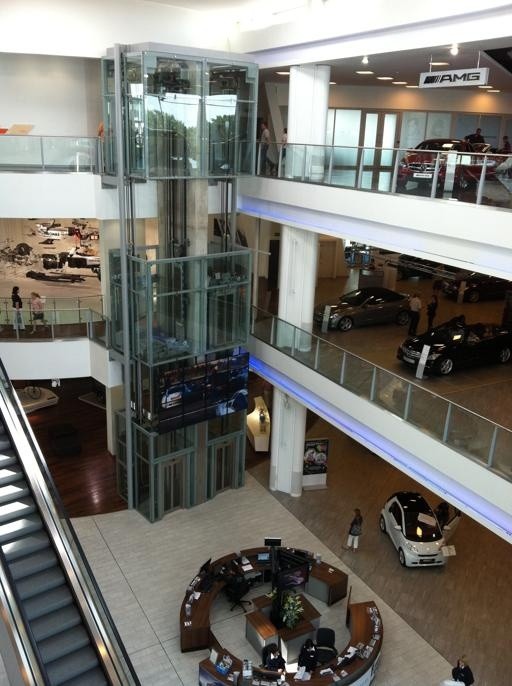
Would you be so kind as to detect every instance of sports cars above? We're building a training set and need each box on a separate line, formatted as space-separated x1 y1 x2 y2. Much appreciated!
397 315 512 375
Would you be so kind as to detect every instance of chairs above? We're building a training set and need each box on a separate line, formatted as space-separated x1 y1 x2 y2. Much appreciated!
262 643 277 666
314 628 338 665
224 580 252 612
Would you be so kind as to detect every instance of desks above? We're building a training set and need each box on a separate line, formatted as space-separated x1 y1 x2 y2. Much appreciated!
245 594 323 665
179 547 348 652
246 395 271 451
198 601 384 686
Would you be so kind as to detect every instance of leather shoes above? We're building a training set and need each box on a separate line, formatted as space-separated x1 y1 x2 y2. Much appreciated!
411 334 416 337
13 328 16 331
20 328 27 331
408 333 411 336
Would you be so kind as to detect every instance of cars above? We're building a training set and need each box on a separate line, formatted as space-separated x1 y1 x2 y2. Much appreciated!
380 491 465 567
434 272 512 303
313 287 410 331
396 139 495 185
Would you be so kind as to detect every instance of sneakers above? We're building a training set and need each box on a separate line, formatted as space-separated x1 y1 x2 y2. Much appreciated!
343 545 349 550
351 548 358 552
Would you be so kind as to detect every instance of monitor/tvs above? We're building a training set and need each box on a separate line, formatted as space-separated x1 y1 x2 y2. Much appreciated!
201 559 211 575
279 564 309 588
264 538 282 547
258 552 271 562
276 547 311 571
338 654 358 668
253 670 281 682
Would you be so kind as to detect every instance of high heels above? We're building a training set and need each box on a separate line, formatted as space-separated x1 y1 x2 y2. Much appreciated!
44 319 48 327
30 329 37 334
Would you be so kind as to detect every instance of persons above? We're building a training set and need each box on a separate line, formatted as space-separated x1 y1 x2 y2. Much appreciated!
297 637 319 676
10 286 26 331
342 509 362 553
448 659 474 686
499 136 512 154
424 294 439 330
265 642 286 671
255 122 277 174
407 292 424 337
465 127 484 144
278 128 287 163
223 565 246 591
435 502 449 528
27 293 48 334
